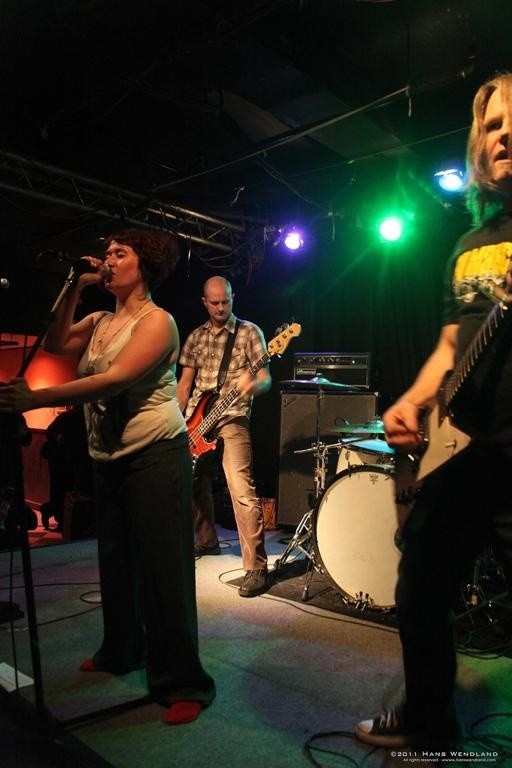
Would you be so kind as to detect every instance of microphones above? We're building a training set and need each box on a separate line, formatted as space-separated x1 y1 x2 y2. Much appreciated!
53 248 116 287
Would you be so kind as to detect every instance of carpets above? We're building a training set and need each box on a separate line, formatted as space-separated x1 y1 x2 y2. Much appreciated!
219 559 400 633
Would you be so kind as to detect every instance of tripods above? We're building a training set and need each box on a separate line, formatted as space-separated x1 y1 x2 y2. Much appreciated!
274 455 327 600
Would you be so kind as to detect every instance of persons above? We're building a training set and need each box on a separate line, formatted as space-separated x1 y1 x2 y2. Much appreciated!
39 403 95 532
350 68 512 749
175 274 274 598
0 221 218 727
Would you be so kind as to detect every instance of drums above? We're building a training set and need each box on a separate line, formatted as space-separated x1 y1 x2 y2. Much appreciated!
335 446 393 475
314 467 400 612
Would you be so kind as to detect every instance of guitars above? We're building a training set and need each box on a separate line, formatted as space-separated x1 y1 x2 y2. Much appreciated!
188 322 306 472
396 255 511 529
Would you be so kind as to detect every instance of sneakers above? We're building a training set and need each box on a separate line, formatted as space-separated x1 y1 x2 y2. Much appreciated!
354 708 463 749
238 568 273 598
192 541 222 556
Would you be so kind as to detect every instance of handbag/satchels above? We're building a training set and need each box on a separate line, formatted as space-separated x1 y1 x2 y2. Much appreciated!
260 497 278 532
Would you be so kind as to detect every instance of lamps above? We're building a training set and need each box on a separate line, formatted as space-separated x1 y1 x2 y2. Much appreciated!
379 157 414 246
273 200 305 252
435 153 469 194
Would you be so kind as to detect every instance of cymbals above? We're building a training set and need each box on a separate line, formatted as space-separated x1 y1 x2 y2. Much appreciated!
350 439 394 456
277 377 360 390
328 421 387 441
